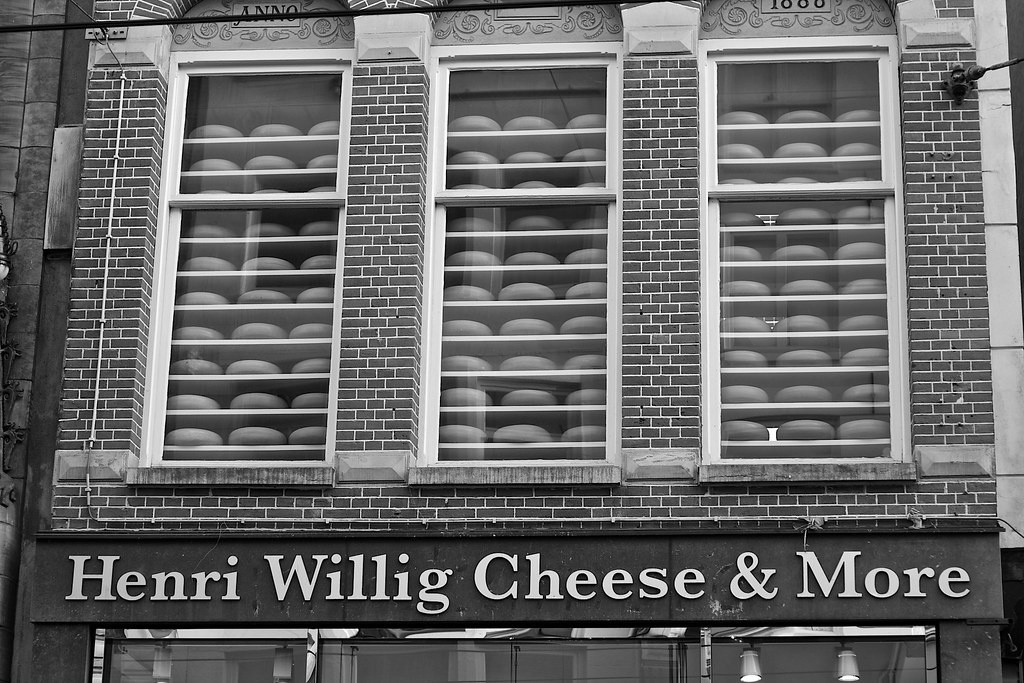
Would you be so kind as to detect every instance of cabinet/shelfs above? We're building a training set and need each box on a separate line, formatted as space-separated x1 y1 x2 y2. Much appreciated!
717 121 891 456
438 126 608 459
163 134 339 459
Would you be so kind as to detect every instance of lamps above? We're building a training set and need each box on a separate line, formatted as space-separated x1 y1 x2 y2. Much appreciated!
152 643 173 683
273 648 294 683
740 643 762 683
836 641 861 681
